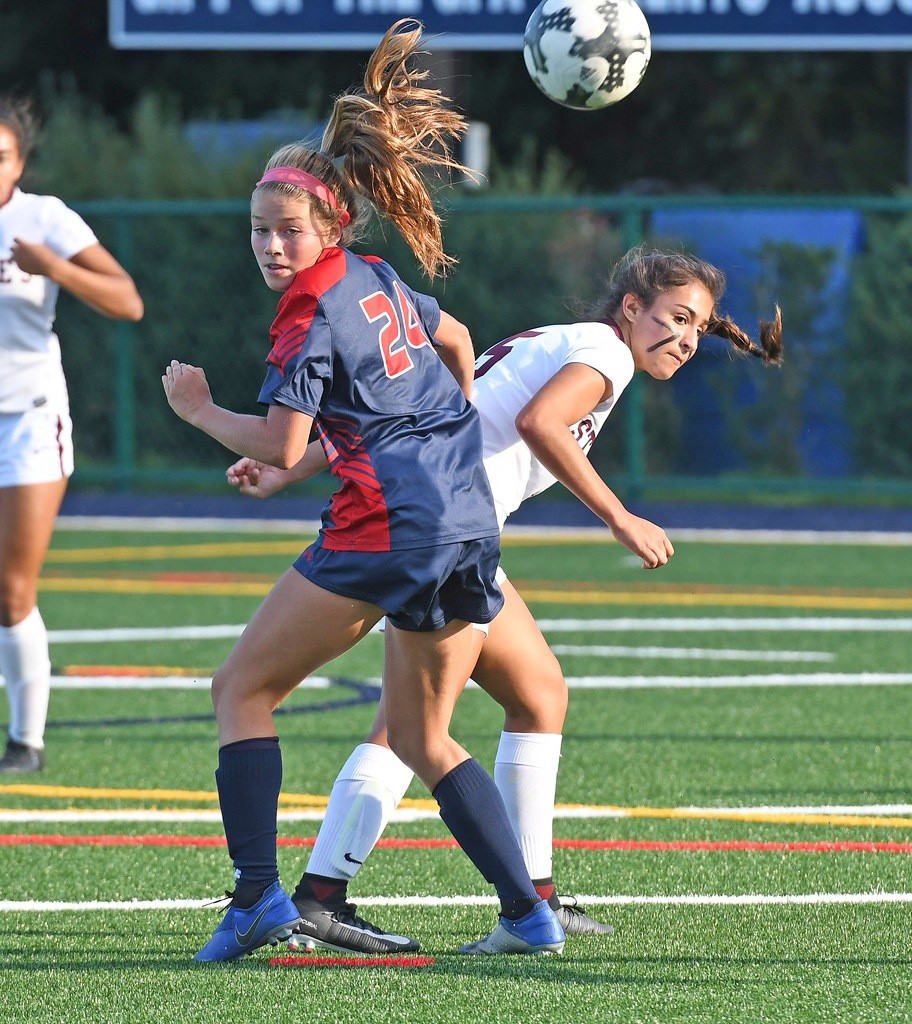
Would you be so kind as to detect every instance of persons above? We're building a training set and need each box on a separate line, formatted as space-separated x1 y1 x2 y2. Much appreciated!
285 250 782 957
0 95 146 774
161 18 562 971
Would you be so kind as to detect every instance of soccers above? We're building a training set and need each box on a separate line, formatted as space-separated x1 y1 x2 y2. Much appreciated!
521 0 653 112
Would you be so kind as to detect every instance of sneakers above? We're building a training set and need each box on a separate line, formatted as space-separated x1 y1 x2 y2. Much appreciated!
194 881 301 962
547 901 612 936
286 880 419 955
459 901 564 958
0 734 44 776
